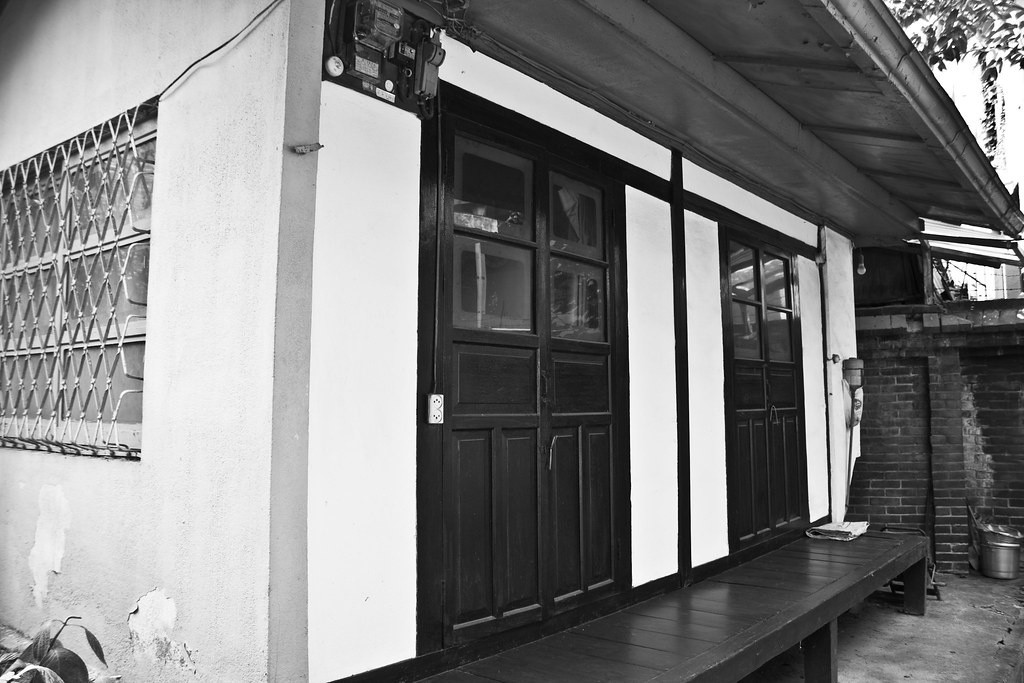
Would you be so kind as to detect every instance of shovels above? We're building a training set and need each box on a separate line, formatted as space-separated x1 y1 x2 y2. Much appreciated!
968 510 982 571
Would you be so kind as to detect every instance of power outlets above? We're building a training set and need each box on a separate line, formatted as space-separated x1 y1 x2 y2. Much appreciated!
427 394 444 424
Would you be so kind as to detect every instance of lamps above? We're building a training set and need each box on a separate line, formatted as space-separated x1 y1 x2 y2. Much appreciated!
843 357 864 391
852 247 866 275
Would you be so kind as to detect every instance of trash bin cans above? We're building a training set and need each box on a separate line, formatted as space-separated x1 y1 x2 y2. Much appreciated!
977 523 1024 580
